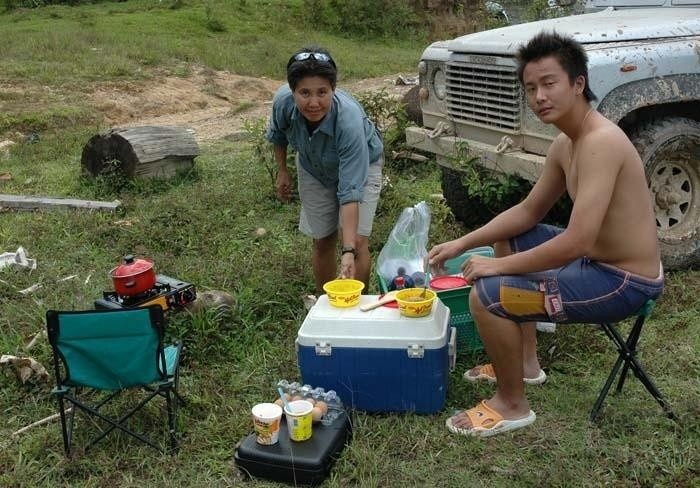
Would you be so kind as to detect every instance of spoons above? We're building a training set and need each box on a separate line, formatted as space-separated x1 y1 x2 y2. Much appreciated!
420 262 430 298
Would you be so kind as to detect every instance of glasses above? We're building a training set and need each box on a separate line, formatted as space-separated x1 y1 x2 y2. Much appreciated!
287 51 338 68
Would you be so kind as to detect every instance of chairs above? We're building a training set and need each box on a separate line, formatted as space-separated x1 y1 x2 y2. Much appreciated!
40 302 191 456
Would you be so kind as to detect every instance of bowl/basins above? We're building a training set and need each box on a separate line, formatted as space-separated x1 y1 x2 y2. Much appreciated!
397 287 436 317
322 279 367 305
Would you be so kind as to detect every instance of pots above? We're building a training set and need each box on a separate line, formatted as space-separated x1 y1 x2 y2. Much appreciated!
109 253 157 295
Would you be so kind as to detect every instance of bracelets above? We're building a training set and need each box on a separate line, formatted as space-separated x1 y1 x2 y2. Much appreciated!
341 246 356 255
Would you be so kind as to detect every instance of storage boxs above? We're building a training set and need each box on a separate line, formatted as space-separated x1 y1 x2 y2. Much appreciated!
229 402 363 479
377 243 499 362
297 286 465 419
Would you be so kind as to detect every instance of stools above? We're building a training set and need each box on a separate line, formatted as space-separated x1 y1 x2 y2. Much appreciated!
574 269 683 439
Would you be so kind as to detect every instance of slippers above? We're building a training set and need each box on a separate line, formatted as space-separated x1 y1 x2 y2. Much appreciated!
464 360 547 384
444 398 536 437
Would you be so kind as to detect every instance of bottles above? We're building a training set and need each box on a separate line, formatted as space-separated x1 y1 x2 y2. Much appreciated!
395 277 406 290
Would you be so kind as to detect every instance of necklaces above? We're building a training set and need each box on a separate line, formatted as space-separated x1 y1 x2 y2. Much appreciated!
566 108 596 167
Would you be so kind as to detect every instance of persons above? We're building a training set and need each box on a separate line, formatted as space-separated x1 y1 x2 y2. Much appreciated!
420 27 666 441
266 47 386 302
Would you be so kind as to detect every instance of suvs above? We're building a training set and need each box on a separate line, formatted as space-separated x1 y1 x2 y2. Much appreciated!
405 0 700 271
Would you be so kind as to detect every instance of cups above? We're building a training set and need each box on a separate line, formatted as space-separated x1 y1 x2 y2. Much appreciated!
251 401 283 446
284 400 313 441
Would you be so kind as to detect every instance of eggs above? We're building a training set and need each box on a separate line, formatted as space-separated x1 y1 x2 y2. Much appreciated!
276 394 328 420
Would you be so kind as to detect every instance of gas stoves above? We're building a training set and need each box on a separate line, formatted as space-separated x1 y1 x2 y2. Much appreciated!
94 273 198 314
